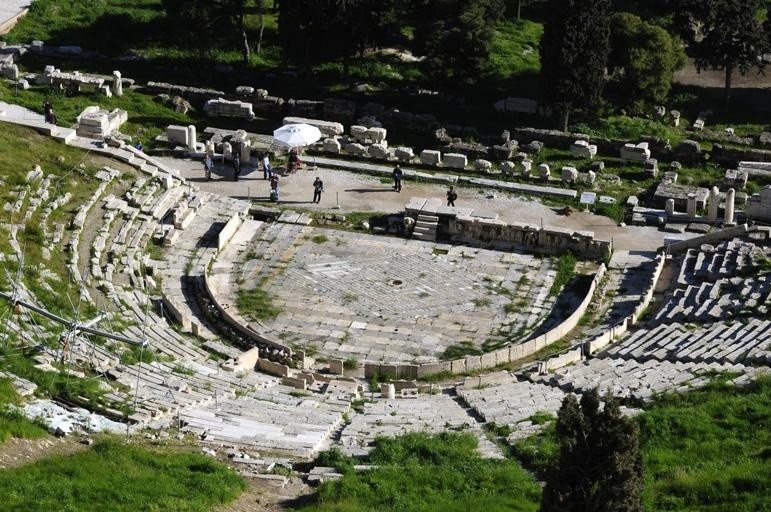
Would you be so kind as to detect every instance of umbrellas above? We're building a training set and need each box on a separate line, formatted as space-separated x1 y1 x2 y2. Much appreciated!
273 123 321 156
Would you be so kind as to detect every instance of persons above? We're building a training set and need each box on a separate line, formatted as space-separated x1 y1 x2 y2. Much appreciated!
286 151 297 173
263 152 271 181
446 186 457 207
393 164 402 193
270 188 279 201
43 100 55 122
232 153 242 182
311 176 325 204
271 171 280 189
203 153 215 180
136 140 146 151
